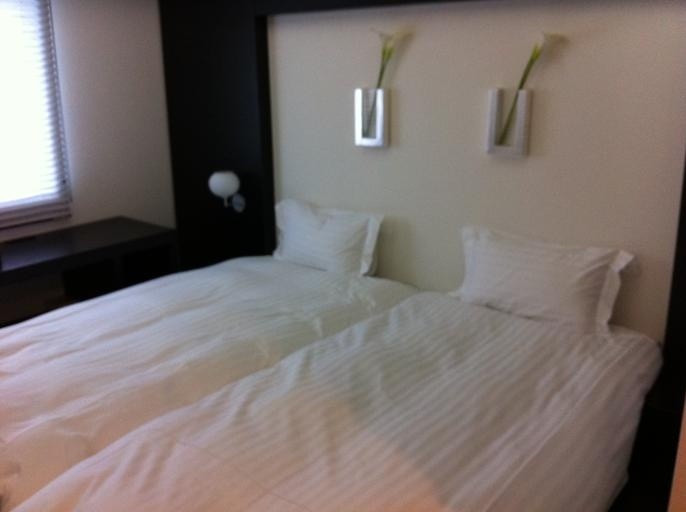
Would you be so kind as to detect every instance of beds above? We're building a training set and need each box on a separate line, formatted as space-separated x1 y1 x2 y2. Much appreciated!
0 256 664 512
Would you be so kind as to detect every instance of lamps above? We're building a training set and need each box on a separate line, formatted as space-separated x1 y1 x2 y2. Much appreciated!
208 170 242 209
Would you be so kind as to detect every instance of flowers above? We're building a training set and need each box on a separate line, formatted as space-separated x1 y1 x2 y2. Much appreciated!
364 28 400 135
497 30 555 145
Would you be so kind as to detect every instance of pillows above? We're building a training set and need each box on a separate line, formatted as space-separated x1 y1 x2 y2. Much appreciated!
271 195 385 273
450 227 635 331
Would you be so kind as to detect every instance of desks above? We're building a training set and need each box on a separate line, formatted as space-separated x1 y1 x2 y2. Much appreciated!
1 215 178 327
